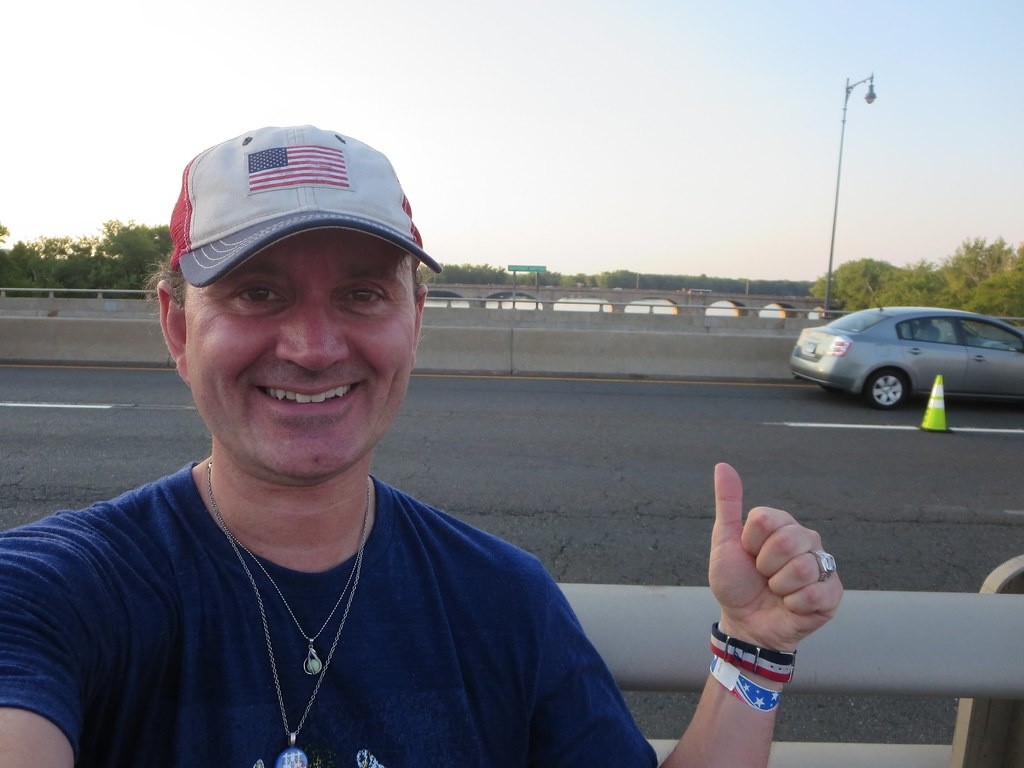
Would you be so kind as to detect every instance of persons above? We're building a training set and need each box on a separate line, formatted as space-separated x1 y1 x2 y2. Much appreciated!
0 114 843 768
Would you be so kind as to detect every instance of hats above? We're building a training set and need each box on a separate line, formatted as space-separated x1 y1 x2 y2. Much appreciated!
169 125 443 288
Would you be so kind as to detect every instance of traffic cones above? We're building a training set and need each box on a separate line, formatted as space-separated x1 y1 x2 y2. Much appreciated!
920 375 954 432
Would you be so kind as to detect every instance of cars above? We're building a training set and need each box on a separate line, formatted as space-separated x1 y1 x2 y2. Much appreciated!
787 305 1024 407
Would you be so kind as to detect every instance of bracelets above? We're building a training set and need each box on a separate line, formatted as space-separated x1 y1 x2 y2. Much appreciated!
708 655 781 712
711 622 796 682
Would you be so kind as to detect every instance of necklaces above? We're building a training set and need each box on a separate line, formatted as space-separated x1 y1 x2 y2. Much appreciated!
225 525 361 676
206 458 371 768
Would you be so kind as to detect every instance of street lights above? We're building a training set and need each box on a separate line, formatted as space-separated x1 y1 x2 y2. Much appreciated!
825 72 879 315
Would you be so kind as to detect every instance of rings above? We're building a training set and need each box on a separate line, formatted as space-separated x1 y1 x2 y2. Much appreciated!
811 546 837 583
